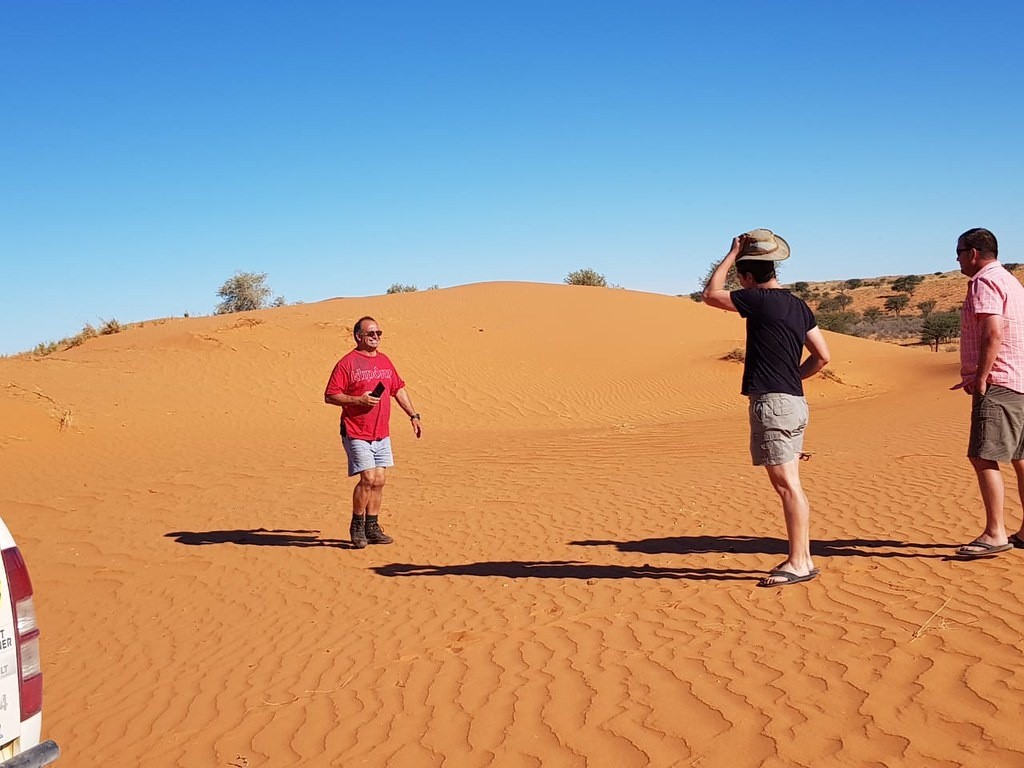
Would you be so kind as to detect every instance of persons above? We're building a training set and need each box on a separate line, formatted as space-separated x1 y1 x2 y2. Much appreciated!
701 228 830 587
325 316 422 549
948 227 1024 556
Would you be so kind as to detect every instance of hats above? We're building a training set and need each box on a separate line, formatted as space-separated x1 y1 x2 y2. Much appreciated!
734 228 790 262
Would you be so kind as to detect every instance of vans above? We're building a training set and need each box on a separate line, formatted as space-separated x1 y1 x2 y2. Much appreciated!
0 516 61 768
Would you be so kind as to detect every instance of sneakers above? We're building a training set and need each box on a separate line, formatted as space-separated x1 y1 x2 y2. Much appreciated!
365 521 393 543
350 519 367 548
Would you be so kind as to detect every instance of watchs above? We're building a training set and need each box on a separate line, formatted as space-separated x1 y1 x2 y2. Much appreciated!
410 413 421 421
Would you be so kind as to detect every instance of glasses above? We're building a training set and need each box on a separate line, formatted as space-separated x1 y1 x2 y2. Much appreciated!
957 248 981 255
360 331 382 337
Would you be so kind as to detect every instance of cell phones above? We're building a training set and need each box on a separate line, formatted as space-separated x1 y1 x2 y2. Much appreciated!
371 381 385 398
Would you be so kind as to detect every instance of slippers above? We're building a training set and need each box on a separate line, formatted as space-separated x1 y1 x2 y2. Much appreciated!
1008 533 1024 548
955 538 1014 555
769 561 820 574
758 567 815 587
